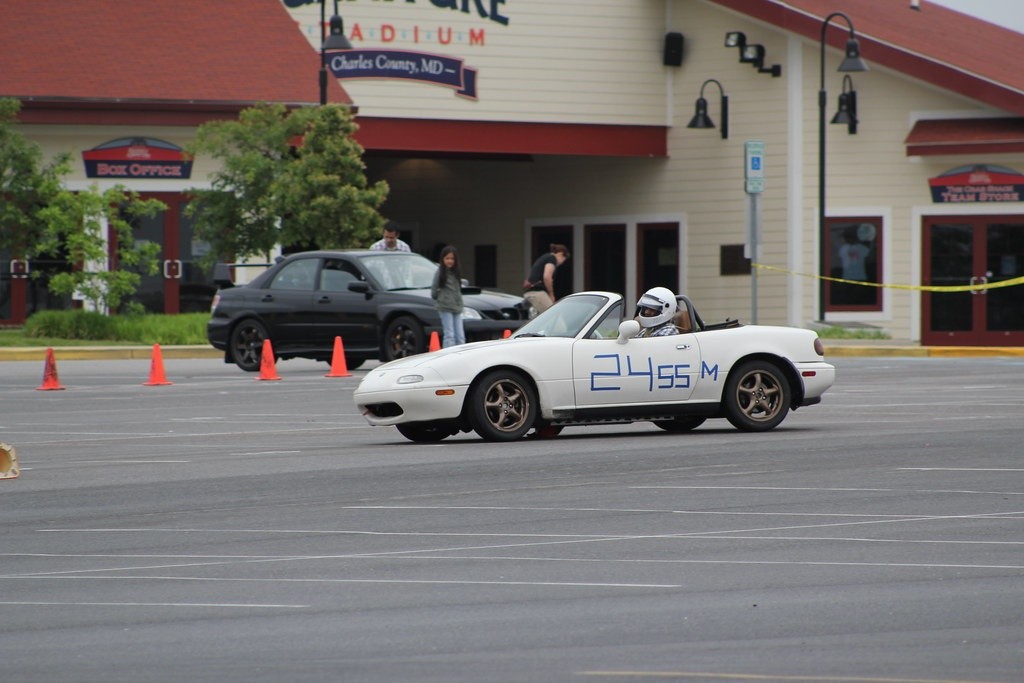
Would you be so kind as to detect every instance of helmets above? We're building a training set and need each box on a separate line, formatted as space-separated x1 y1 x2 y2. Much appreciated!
637 287 677 328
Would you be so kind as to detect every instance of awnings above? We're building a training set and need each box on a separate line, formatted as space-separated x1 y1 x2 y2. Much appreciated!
904 117 1024 156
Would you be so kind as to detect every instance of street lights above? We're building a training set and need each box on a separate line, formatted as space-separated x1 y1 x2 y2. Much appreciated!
817 10 871 321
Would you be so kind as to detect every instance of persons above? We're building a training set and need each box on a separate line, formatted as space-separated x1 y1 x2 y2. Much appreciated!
431 246 466 348
368 220 413 288
523 244 570 313
635 286 680 338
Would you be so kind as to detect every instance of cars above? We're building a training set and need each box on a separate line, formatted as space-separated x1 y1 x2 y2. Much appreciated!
206 248 539 372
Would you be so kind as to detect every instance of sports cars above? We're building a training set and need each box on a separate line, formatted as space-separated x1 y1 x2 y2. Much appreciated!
349 290 838 444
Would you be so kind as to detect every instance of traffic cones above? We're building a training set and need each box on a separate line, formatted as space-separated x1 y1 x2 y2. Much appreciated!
0 442 21 480
35 348 66 390
143 342 174 386
430 331 441 353
253 338 283 381
325 336 353 376
